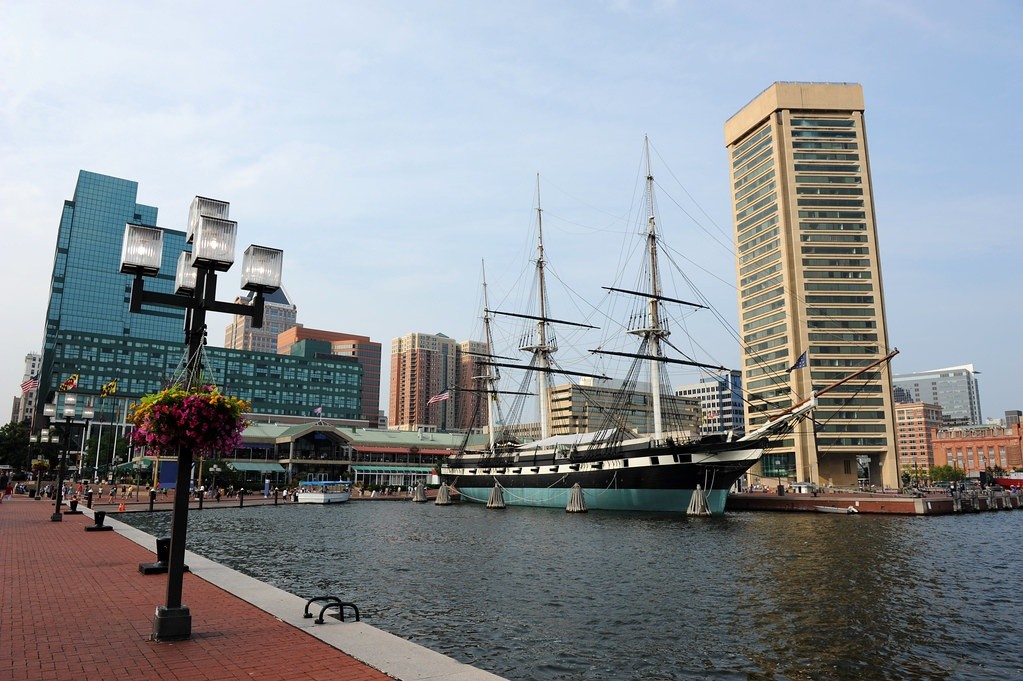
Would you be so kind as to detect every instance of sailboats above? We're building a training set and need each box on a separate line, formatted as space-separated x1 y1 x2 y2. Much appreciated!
441 133 769 512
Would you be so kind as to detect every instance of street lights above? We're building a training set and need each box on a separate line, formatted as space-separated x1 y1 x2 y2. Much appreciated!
133 460 146 502
117 195 282 641
982 456 987 468
29 429 59 500
209 464 221 498
775 460 784 496
43 393 94 522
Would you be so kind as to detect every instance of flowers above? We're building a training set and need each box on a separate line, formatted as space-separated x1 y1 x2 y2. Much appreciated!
124 381 253 460
31 460 50 470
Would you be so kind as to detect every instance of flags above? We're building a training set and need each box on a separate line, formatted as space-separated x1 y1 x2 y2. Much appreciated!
58 373 78 391
99 378 116 397
785 351 807 374
427 386 448 405
20 374 38 393
314 407 322 415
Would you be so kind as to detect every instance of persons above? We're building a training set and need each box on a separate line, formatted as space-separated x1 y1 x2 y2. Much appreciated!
1010 484 1016 493
0 451 448 504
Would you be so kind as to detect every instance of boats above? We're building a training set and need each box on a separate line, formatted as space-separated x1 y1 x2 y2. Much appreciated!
726 491 954 514
298 481 352 503
953 489 1023 511
815 505 858 513
